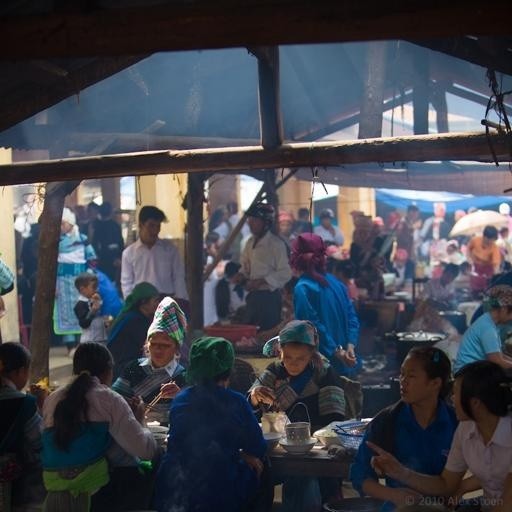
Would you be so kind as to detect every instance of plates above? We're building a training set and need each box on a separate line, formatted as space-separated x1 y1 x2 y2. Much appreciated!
392 291 408 297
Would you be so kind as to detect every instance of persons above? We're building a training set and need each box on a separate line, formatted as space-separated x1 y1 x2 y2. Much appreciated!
247 319 346 511
346 345 483 511
0 198 512 380
110 295 189 426
40 340 158 512
0 341 45 453
149 335 277 512
365 359 512 512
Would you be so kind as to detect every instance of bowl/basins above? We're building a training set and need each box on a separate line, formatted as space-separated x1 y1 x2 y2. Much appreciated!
147 425 169 433
204 323 259 344
152 433 167 446
263 427 341 455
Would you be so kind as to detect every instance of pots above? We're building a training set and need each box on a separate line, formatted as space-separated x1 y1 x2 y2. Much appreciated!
384 328 450 350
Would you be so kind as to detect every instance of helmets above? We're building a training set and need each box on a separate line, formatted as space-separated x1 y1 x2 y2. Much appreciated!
319 208 334 219
245 204 276 225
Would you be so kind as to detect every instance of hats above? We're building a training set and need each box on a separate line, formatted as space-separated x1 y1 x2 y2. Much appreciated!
279 210 293 223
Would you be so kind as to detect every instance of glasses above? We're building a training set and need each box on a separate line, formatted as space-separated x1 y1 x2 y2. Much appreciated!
151 342 173 349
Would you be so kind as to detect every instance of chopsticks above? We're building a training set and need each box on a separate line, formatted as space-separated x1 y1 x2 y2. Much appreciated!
144 379 177 414
255 376 281 433
120 395 161 414
